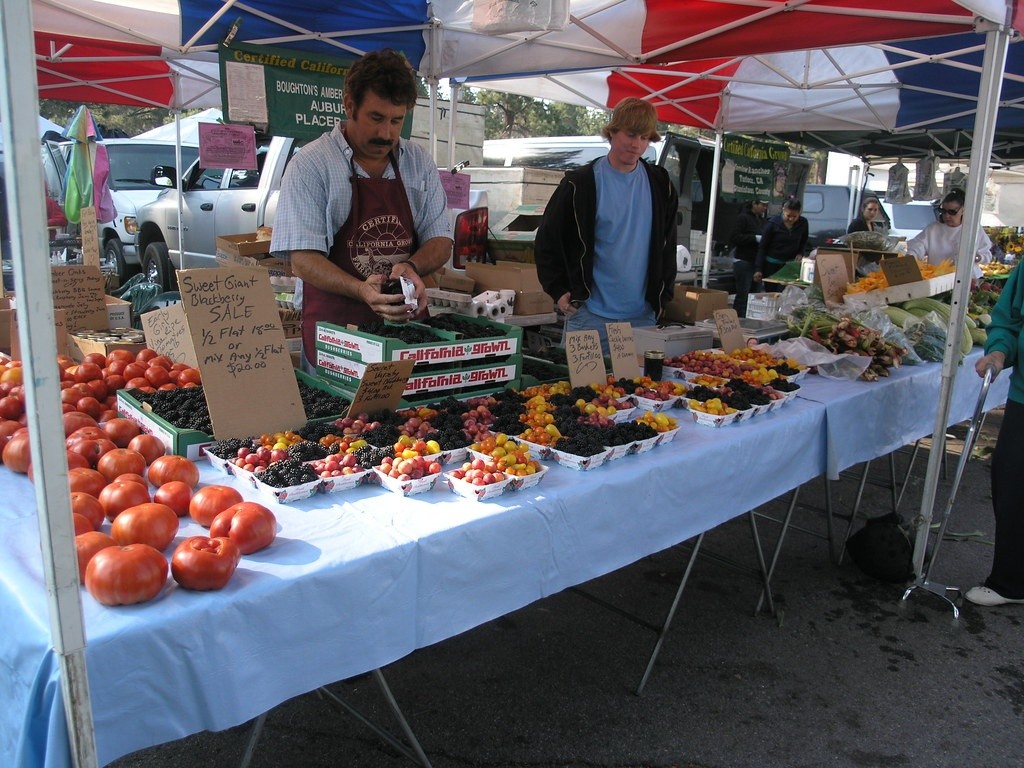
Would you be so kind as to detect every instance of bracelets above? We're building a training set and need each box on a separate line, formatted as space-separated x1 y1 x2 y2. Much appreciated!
398 259 419 275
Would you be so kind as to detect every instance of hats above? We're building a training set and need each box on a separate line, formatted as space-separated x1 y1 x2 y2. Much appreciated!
759 199 770 204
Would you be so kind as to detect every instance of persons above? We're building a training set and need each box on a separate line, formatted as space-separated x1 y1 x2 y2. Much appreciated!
966 257 1023 606
710 197 731 256
905 188 993 266
847 197 878 236
755 196 810 294
269 47 455 379
534 97 679 369
734 200 766 315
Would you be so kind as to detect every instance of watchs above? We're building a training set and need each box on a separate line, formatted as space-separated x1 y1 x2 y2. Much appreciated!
975 253 979 262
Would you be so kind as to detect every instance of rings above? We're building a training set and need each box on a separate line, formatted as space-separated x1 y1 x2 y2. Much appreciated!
565 304 571 311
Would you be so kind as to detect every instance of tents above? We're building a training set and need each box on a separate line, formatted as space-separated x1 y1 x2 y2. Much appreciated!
0 0 1024 768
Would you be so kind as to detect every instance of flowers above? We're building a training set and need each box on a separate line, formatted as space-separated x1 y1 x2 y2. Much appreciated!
982 226 1024 266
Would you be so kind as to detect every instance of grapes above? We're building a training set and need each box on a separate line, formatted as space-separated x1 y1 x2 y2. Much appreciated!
233 387 670 486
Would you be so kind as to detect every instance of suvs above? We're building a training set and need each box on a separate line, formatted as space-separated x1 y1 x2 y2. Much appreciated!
0 138 200 290
800 182 939 257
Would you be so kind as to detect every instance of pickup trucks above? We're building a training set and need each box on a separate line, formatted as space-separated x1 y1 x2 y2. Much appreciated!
134 132 490 303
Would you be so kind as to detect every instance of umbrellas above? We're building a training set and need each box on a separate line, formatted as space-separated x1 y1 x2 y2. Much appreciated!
62 105 117 224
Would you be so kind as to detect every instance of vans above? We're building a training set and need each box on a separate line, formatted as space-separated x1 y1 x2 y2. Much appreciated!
482 131 816 295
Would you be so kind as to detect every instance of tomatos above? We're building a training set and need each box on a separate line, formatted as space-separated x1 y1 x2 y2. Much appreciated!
53 347 275 606
261 347 805 476
0 356 35 484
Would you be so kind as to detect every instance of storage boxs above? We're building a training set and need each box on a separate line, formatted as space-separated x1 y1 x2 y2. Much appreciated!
0 226 981 521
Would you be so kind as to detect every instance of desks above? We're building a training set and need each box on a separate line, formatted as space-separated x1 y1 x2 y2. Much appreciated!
0 344 1016 768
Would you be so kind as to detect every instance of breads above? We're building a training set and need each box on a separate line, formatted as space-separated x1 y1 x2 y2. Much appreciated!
256 227 272 241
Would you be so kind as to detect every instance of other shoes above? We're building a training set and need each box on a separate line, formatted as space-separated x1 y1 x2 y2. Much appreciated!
966 584 1024 607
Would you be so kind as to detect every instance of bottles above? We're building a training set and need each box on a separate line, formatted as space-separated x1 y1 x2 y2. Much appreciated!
644 350 665 381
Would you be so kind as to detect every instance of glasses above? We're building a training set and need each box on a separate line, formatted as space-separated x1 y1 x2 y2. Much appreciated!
938 202 963 216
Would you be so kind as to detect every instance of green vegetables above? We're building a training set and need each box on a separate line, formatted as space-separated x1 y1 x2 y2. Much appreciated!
788 306 946 382
